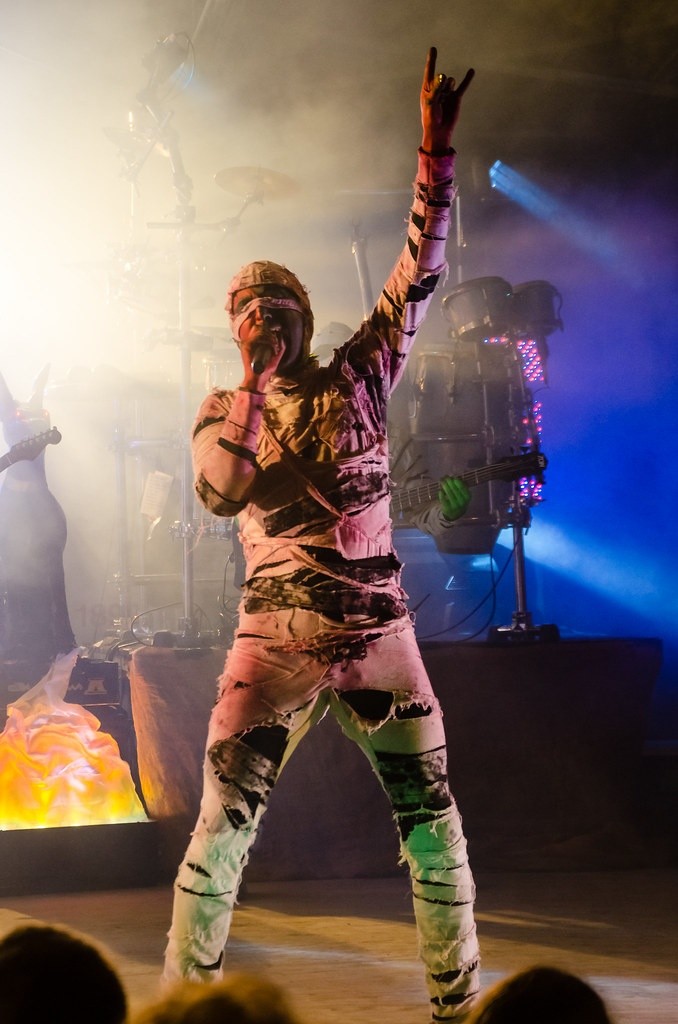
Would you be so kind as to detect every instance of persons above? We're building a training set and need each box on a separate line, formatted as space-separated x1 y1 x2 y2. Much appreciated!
0 921 128 1024
463 963 613 1024
0 365 82 660
160 47 481 1024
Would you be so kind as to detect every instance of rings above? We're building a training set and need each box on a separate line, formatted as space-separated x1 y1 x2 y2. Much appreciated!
438 74 446 84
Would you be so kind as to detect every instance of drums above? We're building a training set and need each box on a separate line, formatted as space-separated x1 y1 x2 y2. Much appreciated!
407 386 485 443
441 273 512 341
413 348 455 389
509 279 565 339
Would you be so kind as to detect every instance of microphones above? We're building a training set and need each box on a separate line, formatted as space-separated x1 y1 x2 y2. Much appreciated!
251 344 273 374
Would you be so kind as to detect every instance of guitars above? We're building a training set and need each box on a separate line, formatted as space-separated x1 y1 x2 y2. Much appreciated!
389 443 548 519
0 425 63 475
